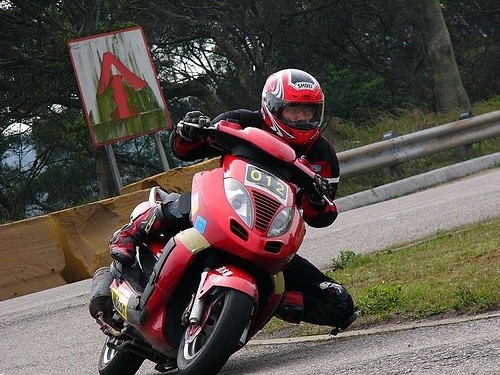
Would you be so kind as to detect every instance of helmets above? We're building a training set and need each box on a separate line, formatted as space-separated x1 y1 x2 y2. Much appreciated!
261 68 325 145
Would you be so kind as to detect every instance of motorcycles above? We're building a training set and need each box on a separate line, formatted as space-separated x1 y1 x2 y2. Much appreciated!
88 121 334 375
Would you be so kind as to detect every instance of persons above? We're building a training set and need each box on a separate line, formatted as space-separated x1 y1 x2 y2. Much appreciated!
108 68 360 336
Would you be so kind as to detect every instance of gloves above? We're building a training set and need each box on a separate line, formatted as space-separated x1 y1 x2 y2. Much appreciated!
182 111 211 141
304 174 332 210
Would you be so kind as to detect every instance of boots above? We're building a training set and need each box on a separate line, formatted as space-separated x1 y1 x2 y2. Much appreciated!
109 204 173 266
270 292 303 323
